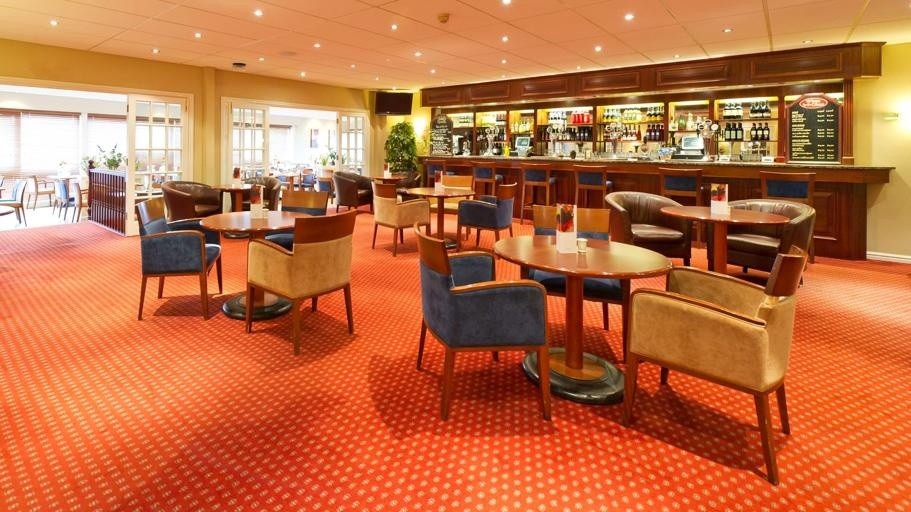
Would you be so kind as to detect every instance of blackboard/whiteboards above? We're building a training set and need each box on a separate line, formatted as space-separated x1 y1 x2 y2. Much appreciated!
785 92 843 165
429 114 454 156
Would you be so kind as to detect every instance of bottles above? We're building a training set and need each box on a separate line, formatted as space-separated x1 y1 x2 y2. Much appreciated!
671 111 701 131
603 124 664 142
510 118 531 133
603 103 665 123
723 99 771 141
452 114 473 128
541 109 593 141
478 112 506 141
479 142 504 155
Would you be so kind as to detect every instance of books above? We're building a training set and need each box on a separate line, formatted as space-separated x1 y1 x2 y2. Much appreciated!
710 181 733 217
434 169 445 195
250 183 265 221
233 167 242 186
554 201 580 255
382 161 391 178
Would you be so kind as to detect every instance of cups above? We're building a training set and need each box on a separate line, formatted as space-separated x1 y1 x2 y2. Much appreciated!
577 238 589 253
263 208 269 218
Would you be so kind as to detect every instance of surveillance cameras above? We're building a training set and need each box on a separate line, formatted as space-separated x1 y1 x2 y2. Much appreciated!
438 13 449 24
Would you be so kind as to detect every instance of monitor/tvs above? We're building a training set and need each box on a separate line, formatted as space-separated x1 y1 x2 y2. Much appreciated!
375 91 413 115
682 135 704 150
515 137 530 149
